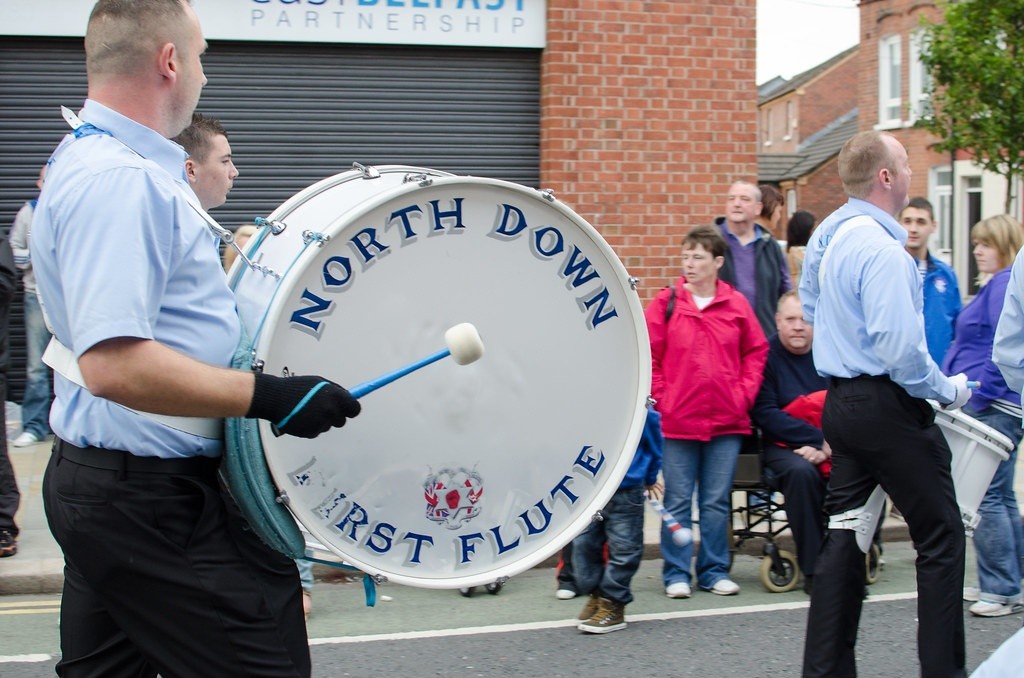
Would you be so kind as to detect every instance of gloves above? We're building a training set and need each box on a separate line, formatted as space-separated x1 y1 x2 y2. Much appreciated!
940 372 972 410
244 372 363 439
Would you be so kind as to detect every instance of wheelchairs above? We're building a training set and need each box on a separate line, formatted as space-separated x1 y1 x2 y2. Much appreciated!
731 425 888 591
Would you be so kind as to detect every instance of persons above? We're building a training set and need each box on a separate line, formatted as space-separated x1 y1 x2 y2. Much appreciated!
714 179 887 597
224 223 314 616
557 396 664 634
644 223 771 599
169 113 239 207
31 1 362 677
798 131 974 677
898 196 961 370
941 215 1023 619
0 162 49 558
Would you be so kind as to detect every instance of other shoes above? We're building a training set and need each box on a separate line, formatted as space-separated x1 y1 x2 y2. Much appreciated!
556 589 576 599
13 431 39 447
302 587 311 615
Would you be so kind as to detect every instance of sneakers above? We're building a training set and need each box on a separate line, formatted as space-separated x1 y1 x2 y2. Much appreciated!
577 588 627 633
666 582 692 598
0 530 16 558
964 586 981 601
710 580 740 596
969 600 1024 618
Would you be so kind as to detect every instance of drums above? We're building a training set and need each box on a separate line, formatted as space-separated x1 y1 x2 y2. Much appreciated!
226 163 654 588
923 399 1016 540
